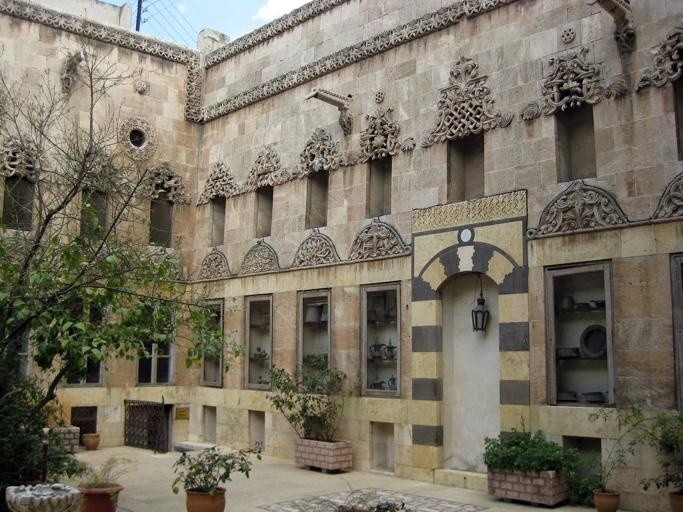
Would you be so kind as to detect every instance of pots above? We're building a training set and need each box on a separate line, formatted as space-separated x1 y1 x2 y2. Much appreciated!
556 295 604 309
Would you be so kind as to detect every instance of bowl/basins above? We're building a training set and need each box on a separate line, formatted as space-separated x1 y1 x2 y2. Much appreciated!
557 390 603 402
555 347 580 358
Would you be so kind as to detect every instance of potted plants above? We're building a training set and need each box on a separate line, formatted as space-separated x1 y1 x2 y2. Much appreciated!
265 357 359 474
575 401 674 511
639 409 683 512
172 438 265 512
481 410 583 506
74 450 138 512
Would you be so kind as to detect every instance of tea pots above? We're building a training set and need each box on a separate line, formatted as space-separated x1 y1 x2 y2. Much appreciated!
368 337 396 390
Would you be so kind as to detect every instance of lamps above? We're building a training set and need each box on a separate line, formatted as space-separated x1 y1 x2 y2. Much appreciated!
468 271 490 335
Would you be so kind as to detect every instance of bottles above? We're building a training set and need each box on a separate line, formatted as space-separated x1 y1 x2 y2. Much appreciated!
253 346 265 358
256 375 262 384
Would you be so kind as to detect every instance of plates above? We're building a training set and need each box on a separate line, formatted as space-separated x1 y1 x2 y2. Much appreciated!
580 325 605 358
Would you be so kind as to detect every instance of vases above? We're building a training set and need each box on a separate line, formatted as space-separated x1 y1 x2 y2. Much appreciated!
81 432 100 450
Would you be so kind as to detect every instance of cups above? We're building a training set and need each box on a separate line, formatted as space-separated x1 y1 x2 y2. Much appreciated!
83 433 99 449
